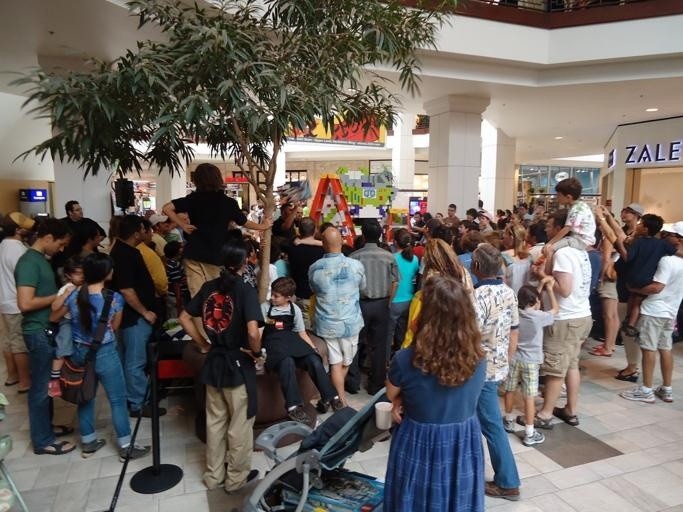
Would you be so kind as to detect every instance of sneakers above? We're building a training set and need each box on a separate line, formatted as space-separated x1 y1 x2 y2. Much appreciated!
620 385 672 403
119 445 151 462
485 481 520 500
626 327 636 337
48 380 62 398
503 407 578 446
289 397 346 423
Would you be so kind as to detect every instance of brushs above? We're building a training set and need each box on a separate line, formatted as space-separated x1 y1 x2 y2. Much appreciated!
309 172 357 249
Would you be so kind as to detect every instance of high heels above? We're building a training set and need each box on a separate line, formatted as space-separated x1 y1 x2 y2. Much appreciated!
616 370 638 382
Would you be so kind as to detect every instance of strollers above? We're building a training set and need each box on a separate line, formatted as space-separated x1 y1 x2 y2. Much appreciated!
243 388 390 510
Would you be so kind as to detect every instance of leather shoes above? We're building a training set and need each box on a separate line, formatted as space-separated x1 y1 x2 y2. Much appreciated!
246 470 258 482
130 404 166 417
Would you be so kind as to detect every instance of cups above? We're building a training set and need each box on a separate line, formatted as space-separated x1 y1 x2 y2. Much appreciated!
374 402 393 430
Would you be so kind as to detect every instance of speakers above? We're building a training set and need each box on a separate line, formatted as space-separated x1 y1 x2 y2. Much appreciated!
117 179 135 207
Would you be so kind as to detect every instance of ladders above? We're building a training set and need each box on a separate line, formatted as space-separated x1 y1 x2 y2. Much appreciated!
385 208 412 248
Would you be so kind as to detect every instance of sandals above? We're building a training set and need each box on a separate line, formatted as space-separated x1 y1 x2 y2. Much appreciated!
35 425 105 458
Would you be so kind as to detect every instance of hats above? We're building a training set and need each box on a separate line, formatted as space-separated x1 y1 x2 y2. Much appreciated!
664 221 683 237
149 214 168 225
480 210 492 220
4 212 35 230
628 203 644 219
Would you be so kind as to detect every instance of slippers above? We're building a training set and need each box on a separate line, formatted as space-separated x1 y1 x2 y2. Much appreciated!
589 345 615 356
5 380 30 393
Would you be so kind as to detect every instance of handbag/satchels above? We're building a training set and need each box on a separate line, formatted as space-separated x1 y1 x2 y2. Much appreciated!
59 356 95 405
